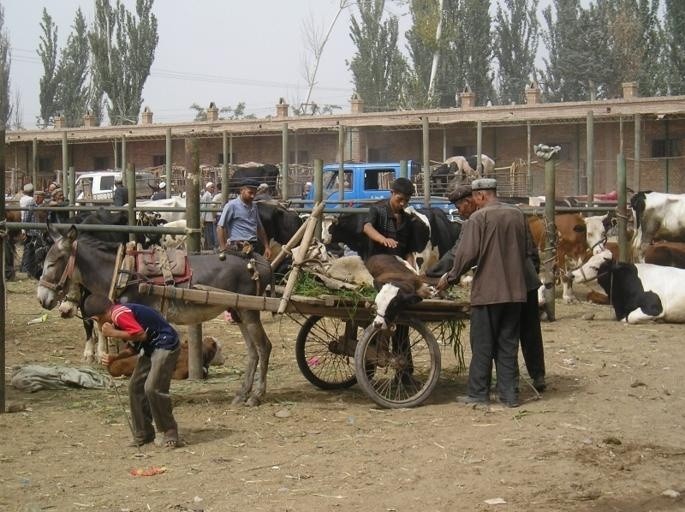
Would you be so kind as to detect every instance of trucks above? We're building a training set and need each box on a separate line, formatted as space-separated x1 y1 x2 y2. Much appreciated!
68 172 168 226
304 158 465 220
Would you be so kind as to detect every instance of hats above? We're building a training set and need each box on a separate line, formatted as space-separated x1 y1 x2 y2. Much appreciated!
471 178 497 190
24 184 33 192
159 182 165 189
240 178 260 188
35 191 47 196
448 185 472 205
206 182 213 187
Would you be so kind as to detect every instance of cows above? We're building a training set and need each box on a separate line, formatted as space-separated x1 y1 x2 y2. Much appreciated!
4 198 303 247
364 254 440 332
526 189 685 325
430 153 496 189
320 205 440 274
106 335 226 381
429 207 461 257
229 163 278 196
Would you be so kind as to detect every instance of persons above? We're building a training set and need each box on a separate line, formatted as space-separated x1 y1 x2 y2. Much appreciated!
437 177 537 410
300 182 313 208
114 179 128 207
426 185 549 395
202 181 222 250
215 179 274 325
20 182 69 271
255 184 270 200
361 178 420 386
83 293 179 450
152 182 166 200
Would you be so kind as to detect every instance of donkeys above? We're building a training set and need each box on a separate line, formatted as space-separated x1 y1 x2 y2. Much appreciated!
58 212 129 364
35 219 276 408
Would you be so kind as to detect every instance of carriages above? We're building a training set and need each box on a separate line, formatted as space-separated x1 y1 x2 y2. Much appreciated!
36 216 470 409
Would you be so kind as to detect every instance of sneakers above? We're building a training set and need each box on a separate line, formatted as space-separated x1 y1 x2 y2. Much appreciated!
456 376 545 407
